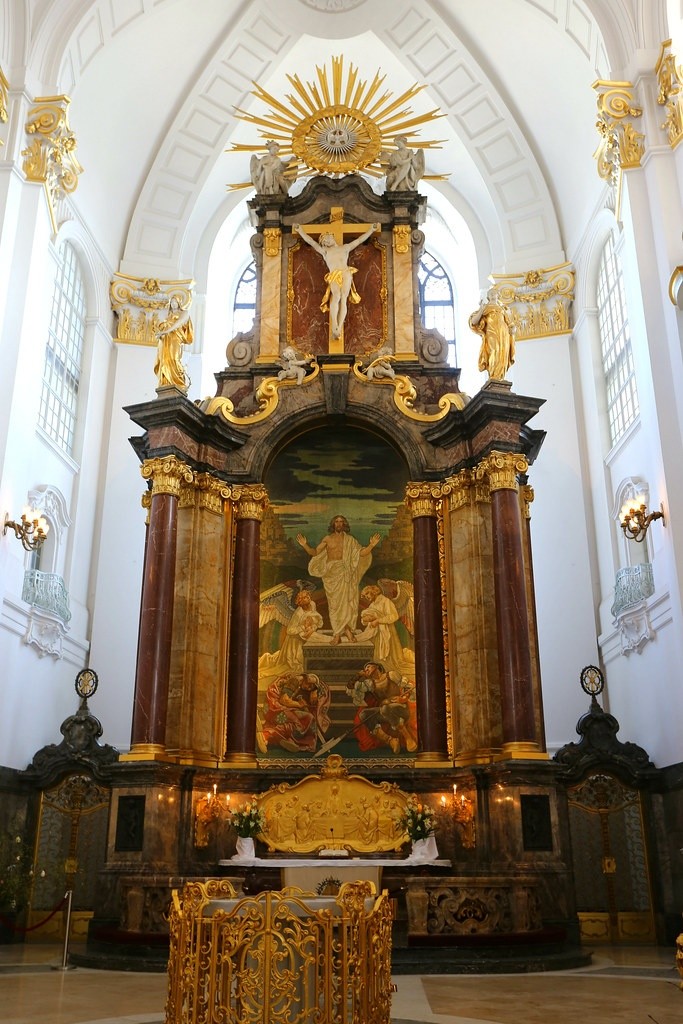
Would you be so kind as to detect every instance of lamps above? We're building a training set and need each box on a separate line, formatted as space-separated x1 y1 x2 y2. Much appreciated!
197 781 230 849
620 499 667 544
3 507 48 552
439 784 477 857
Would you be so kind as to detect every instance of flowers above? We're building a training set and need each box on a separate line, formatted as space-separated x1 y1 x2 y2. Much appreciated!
231 798 268 836
393 794 439 842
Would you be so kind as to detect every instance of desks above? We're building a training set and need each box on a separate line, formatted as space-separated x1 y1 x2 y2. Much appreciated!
189 895 376 1024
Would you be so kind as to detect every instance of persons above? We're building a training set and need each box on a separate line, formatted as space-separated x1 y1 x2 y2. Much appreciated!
259 784 403 846
278 346 311 385
250 141 296 195
469 289 515 379
292 222 378 337
152 295 192 388
366 347 395 379
387 135 424 191
